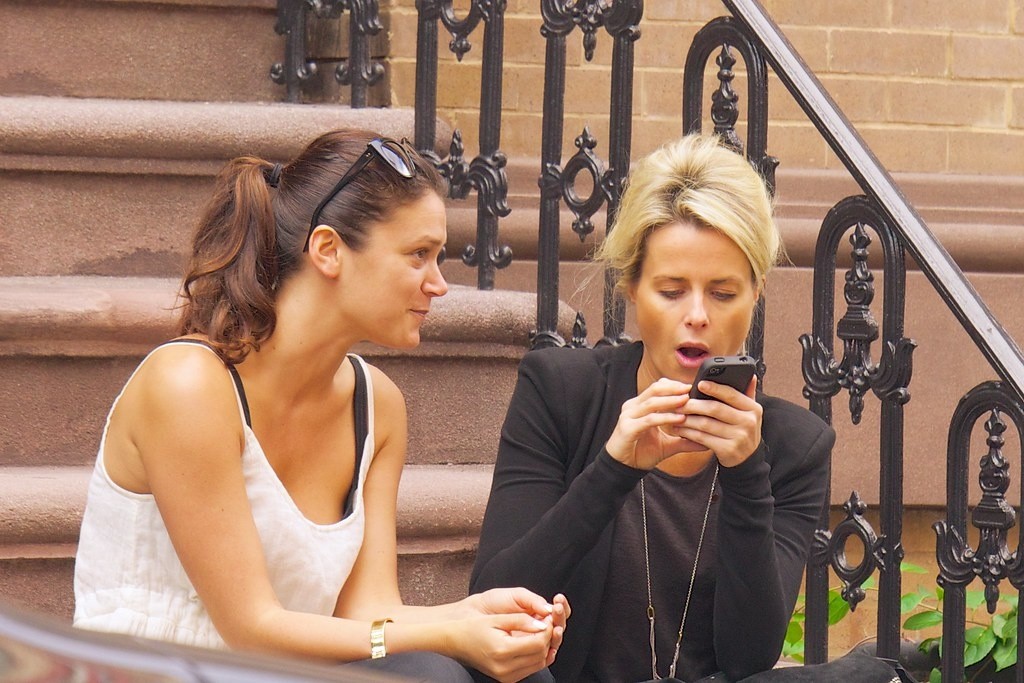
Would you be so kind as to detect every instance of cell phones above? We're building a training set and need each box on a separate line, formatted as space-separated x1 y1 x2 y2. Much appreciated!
686 356 757 421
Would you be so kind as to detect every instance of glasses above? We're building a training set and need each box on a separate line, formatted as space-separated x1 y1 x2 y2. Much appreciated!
303 137 424 252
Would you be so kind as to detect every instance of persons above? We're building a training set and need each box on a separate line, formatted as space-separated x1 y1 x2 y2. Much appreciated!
71 128 573 683
462 134 970 683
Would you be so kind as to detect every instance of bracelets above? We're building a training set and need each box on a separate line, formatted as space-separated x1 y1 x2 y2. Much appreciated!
371 618 393 659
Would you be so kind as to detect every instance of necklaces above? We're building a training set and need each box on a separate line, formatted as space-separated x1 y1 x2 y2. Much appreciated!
639 465 719 679
